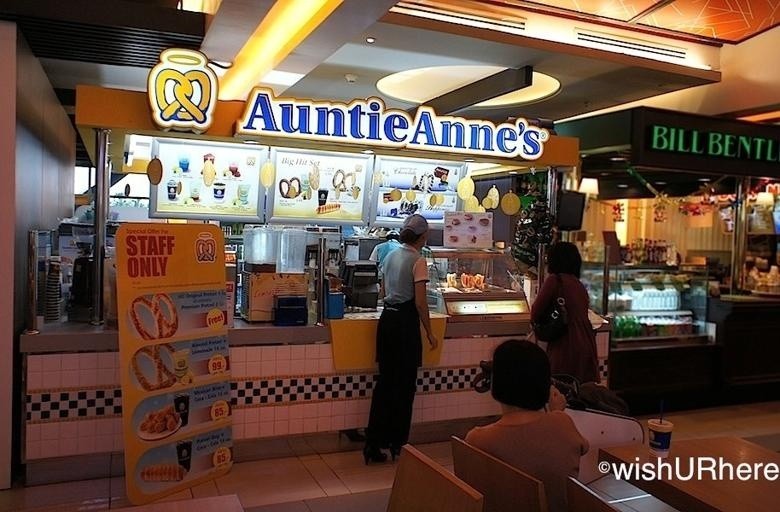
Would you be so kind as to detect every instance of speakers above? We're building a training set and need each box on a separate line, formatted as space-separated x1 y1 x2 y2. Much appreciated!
558 190 586 232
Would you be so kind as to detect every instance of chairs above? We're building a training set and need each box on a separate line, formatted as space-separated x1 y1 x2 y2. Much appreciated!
564 471 619 511
451 433 543 509
380 442 482 511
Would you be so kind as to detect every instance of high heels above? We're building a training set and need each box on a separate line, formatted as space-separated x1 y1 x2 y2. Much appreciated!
389 444 402 462
363 440 387 466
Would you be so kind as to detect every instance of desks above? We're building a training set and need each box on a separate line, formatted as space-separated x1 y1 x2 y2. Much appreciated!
598 433 778 511
110 491 246 511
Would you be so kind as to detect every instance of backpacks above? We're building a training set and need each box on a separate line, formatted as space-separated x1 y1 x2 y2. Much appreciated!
532 270 570 343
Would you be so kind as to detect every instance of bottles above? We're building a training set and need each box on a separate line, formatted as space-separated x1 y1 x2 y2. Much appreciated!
625 237 679 266
587 232 597 262
609 315 694 339
638 288 678 311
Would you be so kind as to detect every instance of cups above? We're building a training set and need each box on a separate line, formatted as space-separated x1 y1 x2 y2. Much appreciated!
164 153 251 205
647 418 674 460
434 167 449 179
325 293 345 318
301 176 328 207
37 255 62 325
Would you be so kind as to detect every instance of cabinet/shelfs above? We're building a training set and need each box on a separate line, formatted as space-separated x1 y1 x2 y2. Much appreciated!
582 260 706 347
422 248 529 322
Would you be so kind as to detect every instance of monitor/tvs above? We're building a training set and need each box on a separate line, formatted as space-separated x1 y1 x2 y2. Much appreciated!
339 260 380 290
772 209 780 234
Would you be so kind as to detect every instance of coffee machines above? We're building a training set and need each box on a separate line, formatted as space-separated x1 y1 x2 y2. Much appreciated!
65 226 99 322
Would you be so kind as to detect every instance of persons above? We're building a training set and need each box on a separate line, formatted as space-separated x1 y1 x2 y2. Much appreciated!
461 338 590 510
530 240 600 383
367 230 400 286
362 213 440 466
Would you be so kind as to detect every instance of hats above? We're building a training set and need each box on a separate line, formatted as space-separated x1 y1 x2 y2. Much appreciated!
489 338 553 414
403 213 428 236
384 229 400 237
